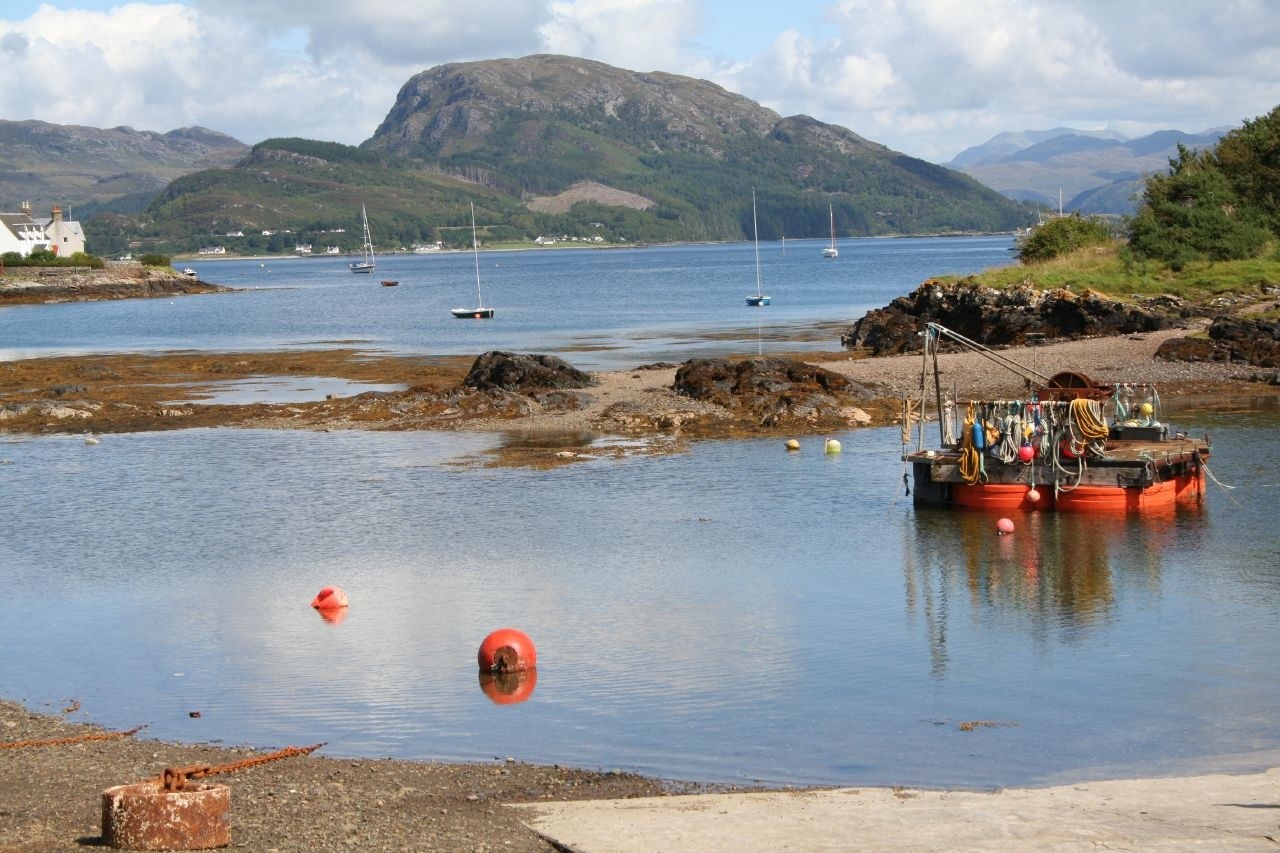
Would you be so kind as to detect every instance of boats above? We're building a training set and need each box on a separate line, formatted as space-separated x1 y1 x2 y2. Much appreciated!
381 281 399 286
183 270 197 276
898 321 1210 516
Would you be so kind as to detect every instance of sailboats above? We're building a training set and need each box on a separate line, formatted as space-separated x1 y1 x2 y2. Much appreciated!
348 202 375 273
822 203 839 257
450 200 494 319
745 186 771 307
